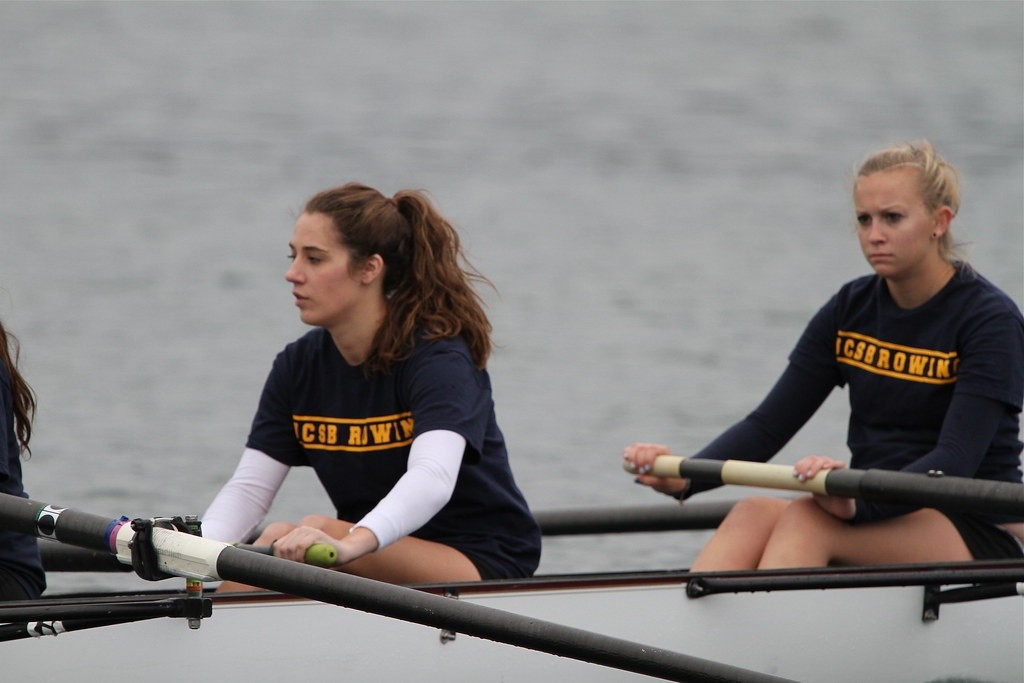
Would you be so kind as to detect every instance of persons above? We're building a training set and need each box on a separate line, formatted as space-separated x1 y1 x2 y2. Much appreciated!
0 316 48 600
621 139 1023 572
199 184 542 603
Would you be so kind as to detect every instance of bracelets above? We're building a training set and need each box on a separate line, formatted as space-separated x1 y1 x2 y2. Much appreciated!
662 477 690 507
